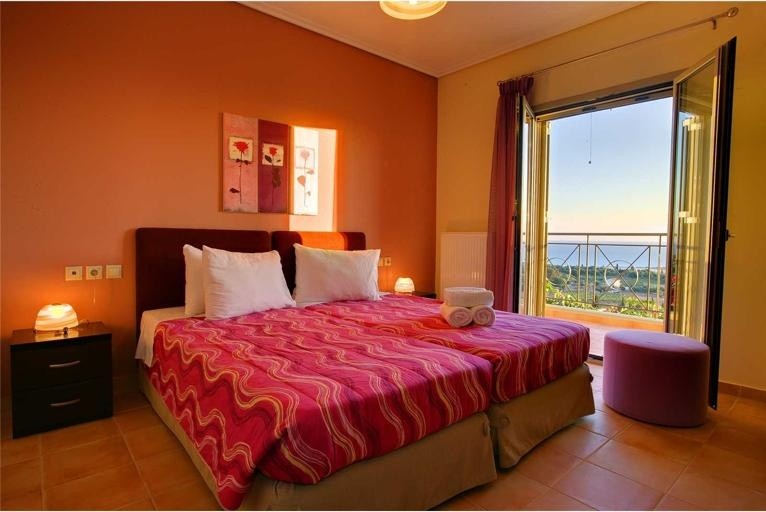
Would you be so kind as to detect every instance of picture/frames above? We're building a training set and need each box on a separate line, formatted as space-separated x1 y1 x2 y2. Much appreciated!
222 109 291 217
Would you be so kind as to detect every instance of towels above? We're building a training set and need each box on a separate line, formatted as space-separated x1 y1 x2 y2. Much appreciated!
441 302 473 327
444 286 495 307
470 304 496 326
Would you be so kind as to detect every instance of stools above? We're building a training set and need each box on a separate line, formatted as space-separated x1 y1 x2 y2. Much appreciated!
604 331 710 428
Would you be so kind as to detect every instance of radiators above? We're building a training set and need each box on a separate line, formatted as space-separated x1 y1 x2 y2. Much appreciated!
439 231 488 300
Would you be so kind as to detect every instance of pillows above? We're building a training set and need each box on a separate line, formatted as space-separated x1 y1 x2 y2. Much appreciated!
201 244 292 322
183 243 205 317
291 243 382 308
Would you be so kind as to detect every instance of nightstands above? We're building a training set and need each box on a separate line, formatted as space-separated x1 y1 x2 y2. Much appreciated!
11 320 114 438
413 290 437 299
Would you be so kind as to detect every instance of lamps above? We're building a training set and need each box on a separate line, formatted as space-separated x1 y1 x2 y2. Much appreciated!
33 301 79 334
377 1 449 22
395 277 415 295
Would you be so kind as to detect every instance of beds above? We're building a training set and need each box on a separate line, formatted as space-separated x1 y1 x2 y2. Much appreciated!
132 226 595 510
